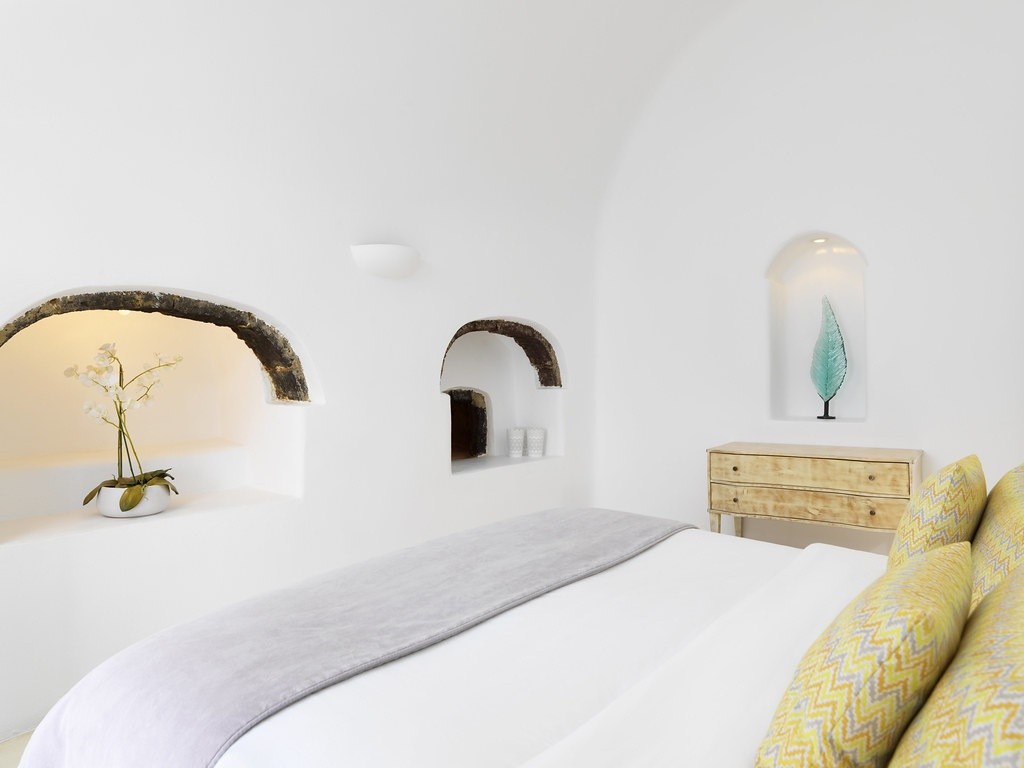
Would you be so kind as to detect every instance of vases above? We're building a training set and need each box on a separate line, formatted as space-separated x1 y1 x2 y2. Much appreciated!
506 426 545 459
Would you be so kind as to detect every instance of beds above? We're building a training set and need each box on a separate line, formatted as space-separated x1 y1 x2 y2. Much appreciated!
20 503 1021 766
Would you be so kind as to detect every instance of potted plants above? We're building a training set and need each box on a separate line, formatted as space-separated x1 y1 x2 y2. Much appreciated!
55 339 186 519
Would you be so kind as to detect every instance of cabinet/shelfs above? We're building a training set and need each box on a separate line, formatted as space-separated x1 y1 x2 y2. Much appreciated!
706 439 924 559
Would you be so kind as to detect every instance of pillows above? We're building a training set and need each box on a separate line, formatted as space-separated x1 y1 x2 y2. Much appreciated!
749 541 981 765
883 456 990 585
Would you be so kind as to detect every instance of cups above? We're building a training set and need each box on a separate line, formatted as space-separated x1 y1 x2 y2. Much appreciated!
507 427 524 457
527 427 545 457
